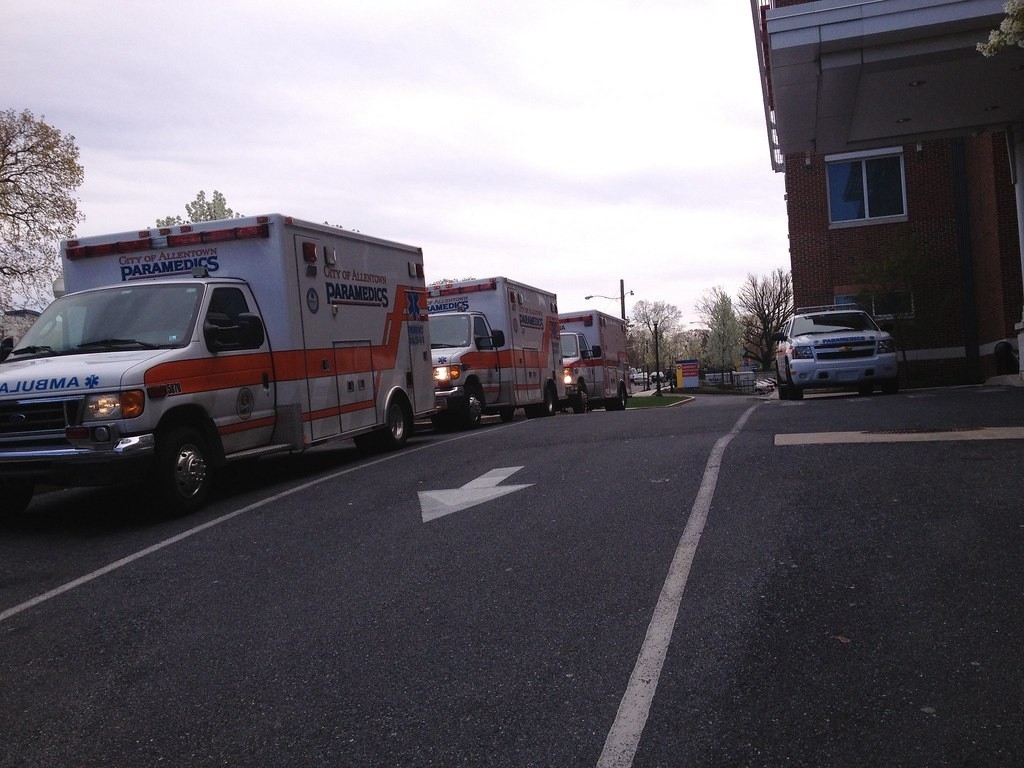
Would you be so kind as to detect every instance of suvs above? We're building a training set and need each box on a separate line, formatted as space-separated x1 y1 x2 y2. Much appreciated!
770 303 901 402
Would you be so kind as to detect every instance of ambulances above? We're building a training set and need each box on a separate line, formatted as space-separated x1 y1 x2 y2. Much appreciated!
0 211 439 511
558 309 633 414
426 275 567 429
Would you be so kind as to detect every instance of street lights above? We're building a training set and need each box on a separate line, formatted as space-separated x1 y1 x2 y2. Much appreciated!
652 315 663 396
584 279 634 320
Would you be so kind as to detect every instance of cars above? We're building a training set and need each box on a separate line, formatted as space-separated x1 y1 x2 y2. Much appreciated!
630 365 666 385
754 377 779 396
